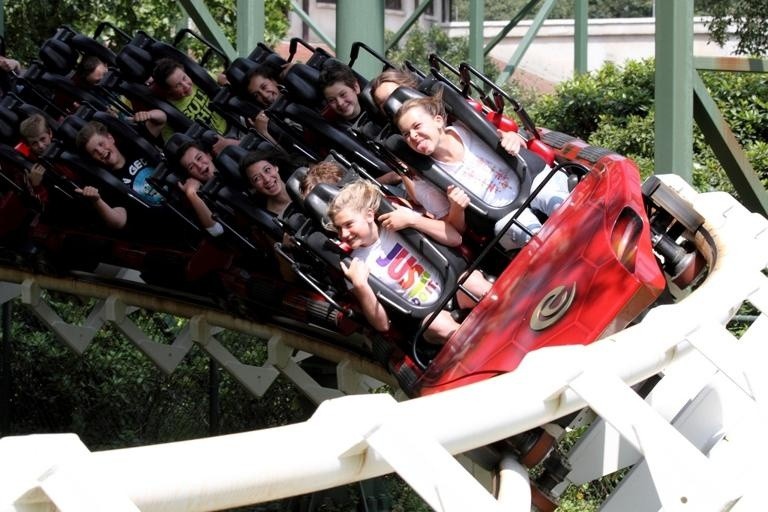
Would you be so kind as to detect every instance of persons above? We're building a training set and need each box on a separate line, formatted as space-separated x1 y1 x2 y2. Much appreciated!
326 180 493 344
370 69 419 124
72 109 169 228
300 161 353 253
0 56 23 77
318 63 407 185
242 62 297 155
19 113 75 203
238 151 295 222
175 134 242 239
152 59 231 146
78 57 134 118
393 96 570 250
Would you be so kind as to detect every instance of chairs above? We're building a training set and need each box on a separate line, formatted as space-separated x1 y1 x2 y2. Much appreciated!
263 52 392 174
39 100 162 214
97 30 221 136
290 162 474 345
210 43 307 160
196 127 294 236
272 148 350 236
375 78 555 245
1 91 61 187
22 28 116 112
145 119 222 215
353 73 483 140
